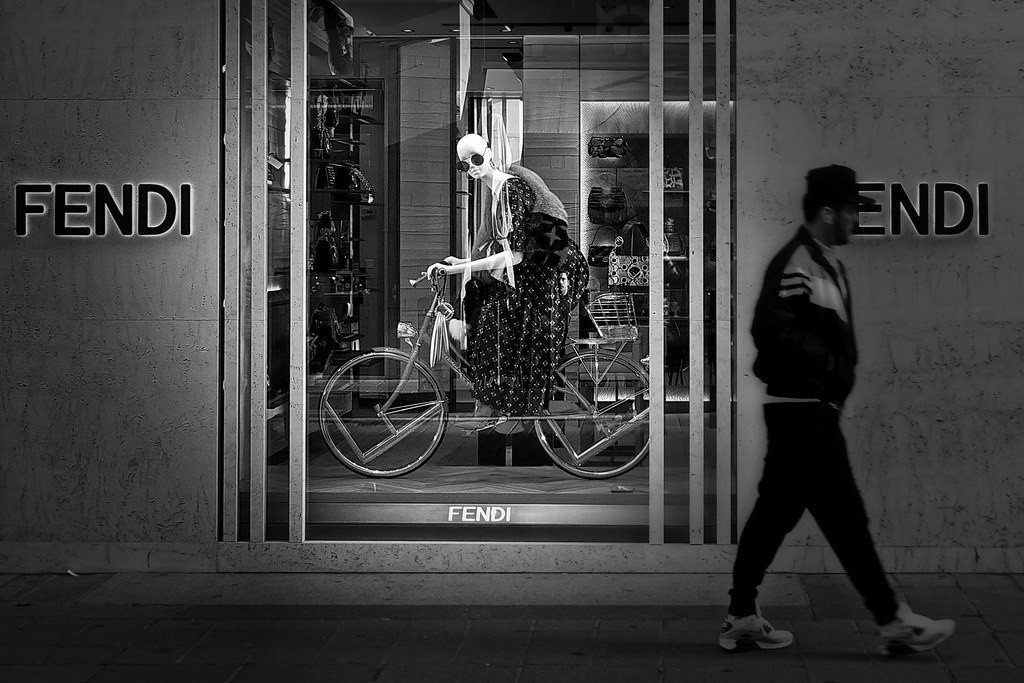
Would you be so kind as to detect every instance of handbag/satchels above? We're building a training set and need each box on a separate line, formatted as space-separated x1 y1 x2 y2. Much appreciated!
307 90 376 352
264 152 292 191
429 268 449 368
586 121 688 318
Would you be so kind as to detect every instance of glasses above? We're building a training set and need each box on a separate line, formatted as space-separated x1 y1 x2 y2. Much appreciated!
457 148 486 173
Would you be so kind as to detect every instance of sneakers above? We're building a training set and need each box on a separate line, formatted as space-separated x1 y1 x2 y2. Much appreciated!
872 602 954 655
719 601 793 650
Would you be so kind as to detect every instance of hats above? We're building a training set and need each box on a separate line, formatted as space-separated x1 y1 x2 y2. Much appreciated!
806 164 875 205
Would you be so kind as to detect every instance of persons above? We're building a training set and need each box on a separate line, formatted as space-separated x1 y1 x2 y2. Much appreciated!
718 166 956 651
428 133 577 424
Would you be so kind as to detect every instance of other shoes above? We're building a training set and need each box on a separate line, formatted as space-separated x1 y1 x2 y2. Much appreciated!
455 408 503 428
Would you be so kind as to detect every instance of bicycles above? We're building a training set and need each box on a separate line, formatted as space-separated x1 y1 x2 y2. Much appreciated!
314 267 655 479
